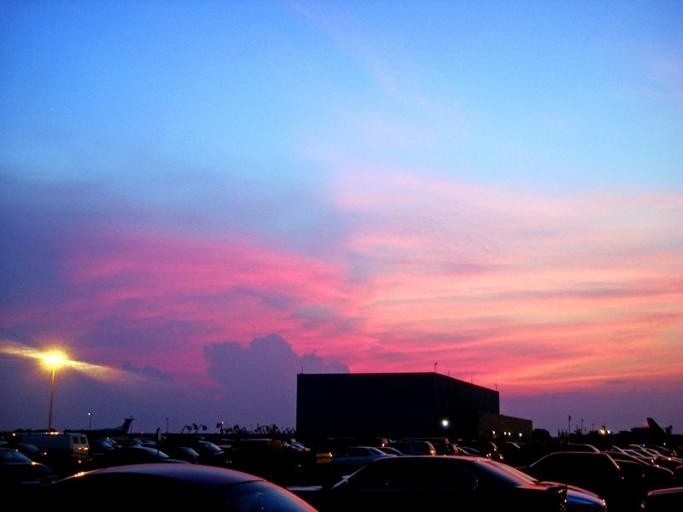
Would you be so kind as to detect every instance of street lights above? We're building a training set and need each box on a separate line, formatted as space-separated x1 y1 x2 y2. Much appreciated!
37 345 68 431
